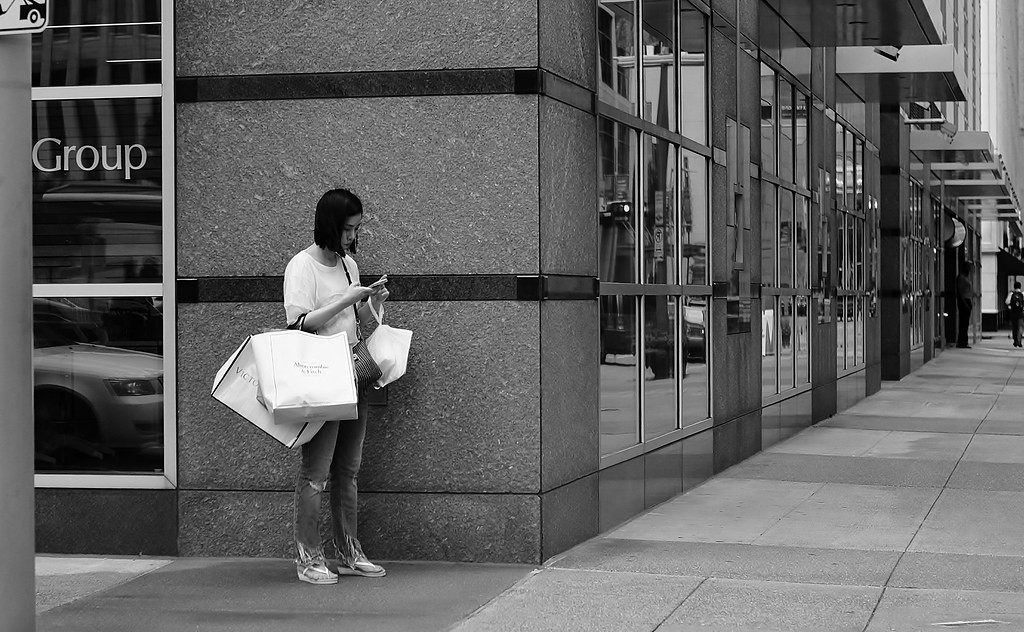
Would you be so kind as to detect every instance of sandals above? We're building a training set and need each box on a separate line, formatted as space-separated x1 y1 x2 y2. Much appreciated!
296 561 338 585
336 556 386 578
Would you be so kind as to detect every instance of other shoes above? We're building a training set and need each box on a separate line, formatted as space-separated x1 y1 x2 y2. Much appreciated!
956 346 971 348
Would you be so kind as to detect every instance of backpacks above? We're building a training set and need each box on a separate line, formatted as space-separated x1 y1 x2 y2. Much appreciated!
1010 291 1024 314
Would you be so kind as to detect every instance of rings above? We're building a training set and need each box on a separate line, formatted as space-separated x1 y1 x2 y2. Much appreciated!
381 289 383 293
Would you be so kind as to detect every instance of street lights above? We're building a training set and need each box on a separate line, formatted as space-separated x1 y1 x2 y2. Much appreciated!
684 218 694 306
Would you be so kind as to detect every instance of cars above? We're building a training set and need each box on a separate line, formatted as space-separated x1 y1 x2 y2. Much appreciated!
598 295 706 378
31 311 164 473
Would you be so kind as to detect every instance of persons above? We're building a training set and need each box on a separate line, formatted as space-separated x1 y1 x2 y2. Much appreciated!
955 261 975 348
781 297 793 316
283 188 389 584
1005 282 1024 347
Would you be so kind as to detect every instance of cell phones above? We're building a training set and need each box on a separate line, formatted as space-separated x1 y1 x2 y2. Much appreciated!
369 279 388 288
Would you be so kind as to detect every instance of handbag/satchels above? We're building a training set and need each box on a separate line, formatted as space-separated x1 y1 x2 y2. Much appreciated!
249 314 359 422
211 314 332 450
256 329 286 411
367 296 413 391
352 340 382 386
